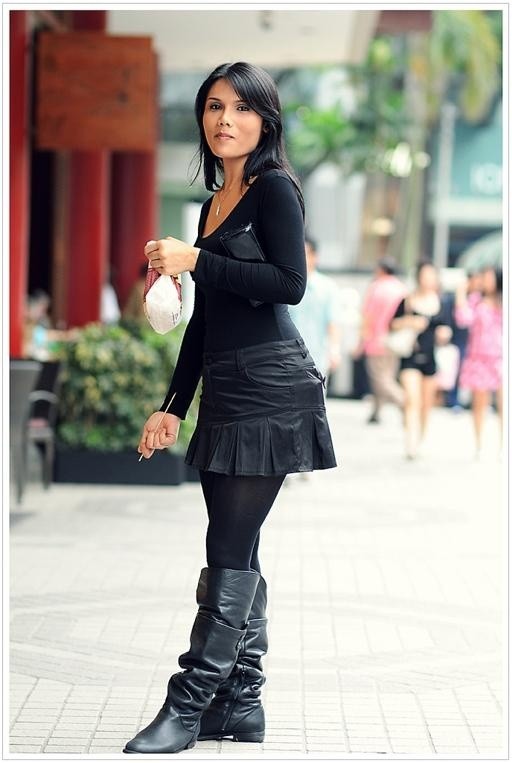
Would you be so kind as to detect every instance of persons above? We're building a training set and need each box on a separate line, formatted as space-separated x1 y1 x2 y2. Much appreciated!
352 256 453 462
455 261 503 463
286 235 339 402
23 251 124 434
116 61 339 753
124 253 152 323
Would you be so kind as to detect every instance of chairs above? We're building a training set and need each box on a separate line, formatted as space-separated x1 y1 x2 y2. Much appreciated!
9 354 61 512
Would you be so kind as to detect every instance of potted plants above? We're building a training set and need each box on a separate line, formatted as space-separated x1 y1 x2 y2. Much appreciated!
52 316 192 487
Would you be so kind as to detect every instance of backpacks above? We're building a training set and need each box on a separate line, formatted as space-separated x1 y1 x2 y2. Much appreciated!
381 327 416 359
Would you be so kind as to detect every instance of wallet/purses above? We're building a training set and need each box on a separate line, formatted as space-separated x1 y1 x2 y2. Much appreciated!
219 223 270 307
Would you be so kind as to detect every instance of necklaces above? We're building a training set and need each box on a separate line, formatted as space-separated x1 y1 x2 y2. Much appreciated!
213 182 236 217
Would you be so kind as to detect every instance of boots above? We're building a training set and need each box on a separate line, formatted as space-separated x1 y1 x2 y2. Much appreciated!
123 567 268 753
